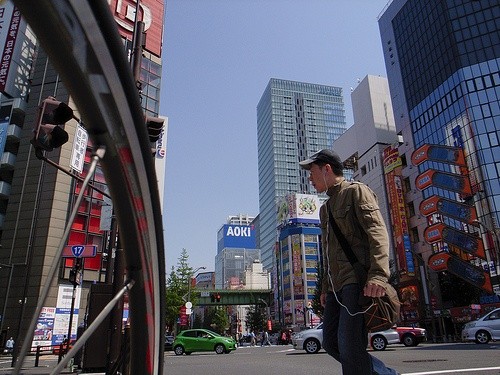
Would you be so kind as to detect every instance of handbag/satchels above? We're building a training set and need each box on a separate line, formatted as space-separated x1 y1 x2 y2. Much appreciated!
357 281 400 332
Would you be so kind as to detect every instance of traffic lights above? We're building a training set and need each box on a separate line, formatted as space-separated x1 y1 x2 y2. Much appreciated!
211 293 221 303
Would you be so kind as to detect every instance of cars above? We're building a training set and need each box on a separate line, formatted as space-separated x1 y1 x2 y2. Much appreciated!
292 322 400 353
461 307 500 344
393 324 426 346
172 329 236 355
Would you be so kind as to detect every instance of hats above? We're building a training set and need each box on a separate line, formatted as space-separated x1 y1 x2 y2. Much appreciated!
299 148 344 170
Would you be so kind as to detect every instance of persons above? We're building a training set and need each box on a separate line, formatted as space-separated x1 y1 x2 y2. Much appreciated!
249 330 293 347
299 149 401 375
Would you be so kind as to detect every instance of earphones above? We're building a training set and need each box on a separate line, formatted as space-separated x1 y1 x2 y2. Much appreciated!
326 168 328 171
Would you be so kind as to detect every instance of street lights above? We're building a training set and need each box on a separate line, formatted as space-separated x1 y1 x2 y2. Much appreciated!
187 266 207 301
